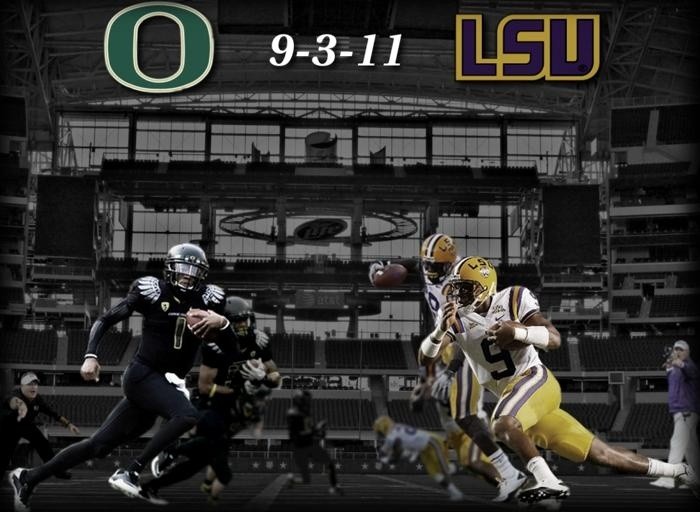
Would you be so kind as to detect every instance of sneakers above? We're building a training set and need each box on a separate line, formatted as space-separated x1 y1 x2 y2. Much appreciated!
201 484 212 493
519 480 570 502
492 473 528 503
108 469 149 501
140 486 169 506
9 468 37 512
677 466 700 500
151 456 164 478
650 478 673 489
679 484 688 489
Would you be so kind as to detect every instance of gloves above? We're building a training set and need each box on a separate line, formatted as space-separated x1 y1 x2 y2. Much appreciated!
369 260 390 284
242 381 261 396
431 369 455 404
240 358 267 383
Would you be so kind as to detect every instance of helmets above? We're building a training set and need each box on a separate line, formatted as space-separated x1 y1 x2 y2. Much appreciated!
223 296 257 341
165 243 209 295
373 417 393 439
421 234 456 281
293 391 312 409
449 256 497 312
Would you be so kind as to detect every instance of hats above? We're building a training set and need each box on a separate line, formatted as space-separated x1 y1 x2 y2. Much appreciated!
21 372 40 386
674 341 689 351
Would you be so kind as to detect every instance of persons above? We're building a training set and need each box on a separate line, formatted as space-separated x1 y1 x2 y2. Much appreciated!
139 295 280 505
1 373 82 481
418 257 699 506
285 389 343 496
10 243 240 508
202 395 268 509
650 339 700 489
373 415 465 502
368 234 527 504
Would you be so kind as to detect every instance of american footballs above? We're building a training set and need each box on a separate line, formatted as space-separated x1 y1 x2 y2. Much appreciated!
374 264 408 286
244 360 267 376
486 321 532 350
187 309 218 341
265 371 281 387
